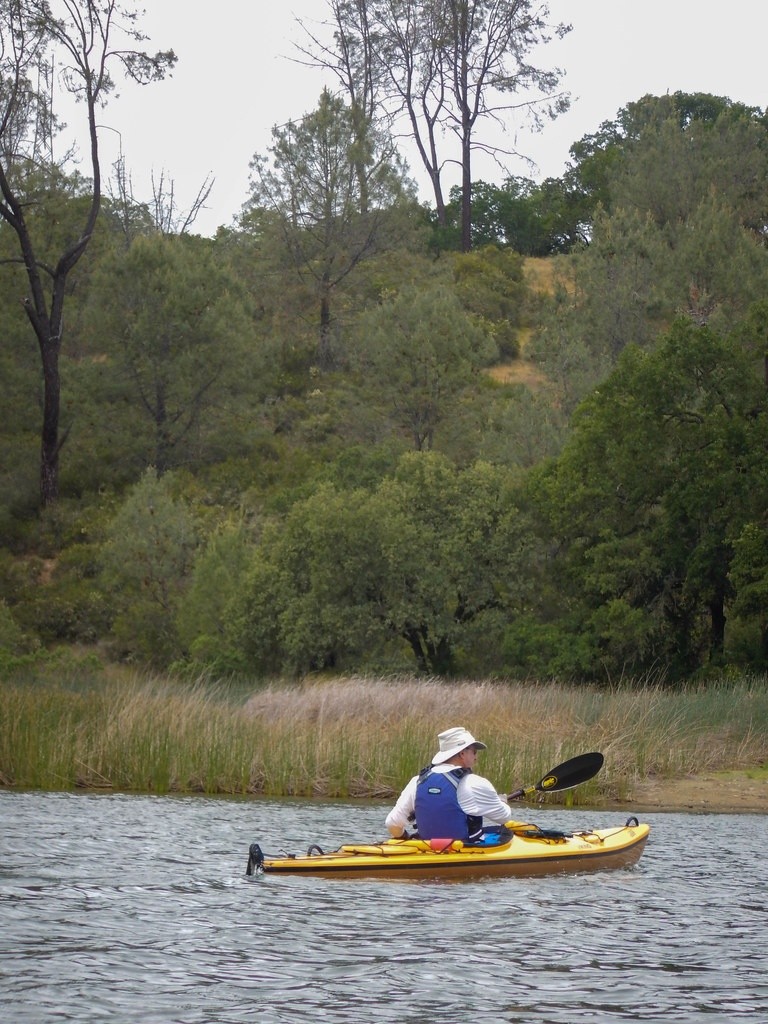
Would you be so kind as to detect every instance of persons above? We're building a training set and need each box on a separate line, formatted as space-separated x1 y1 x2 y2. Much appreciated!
384 724 511 845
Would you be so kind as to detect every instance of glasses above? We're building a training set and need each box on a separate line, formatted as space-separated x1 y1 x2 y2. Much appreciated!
466 747 478 755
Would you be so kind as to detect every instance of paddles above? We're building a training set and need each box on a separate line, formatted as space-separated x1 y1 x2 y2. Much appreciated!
412 750 606 830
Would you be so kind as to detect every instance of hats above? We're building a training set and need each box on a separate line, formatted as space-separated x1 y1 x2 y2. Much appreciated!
432 726 487 764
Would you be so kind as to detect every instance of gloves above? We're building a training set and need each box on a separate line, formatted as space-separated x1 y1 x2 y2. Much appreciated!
394 832 410 840
498 793 508 803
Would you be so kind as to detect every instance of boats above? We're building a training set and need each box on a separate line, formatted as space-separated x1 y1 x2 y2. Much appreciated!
246 816 651 879
